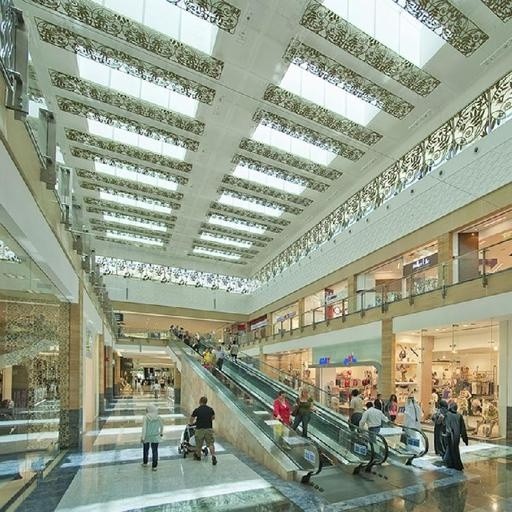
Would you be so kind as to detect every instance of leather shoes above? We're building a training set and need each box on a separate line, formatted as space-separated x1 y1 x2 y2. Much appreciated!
195 455 217 467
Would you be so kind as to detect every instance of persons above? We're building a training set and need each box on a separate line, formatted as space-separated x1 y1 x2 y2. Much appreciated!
134 323 239 399
292 386 314 438
348 389 468 470
190 396 218 465
458 388 472 430
475 403 498 436
273 390 292 451
140 403 164 470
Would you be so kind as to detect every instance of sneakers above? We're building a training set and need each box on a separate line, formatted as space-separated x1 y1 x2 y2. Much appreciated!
143 463 157 470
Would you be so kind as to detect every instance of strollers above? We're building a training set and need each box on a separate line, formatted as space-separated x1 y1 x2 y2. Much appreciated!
178 422 209 458
